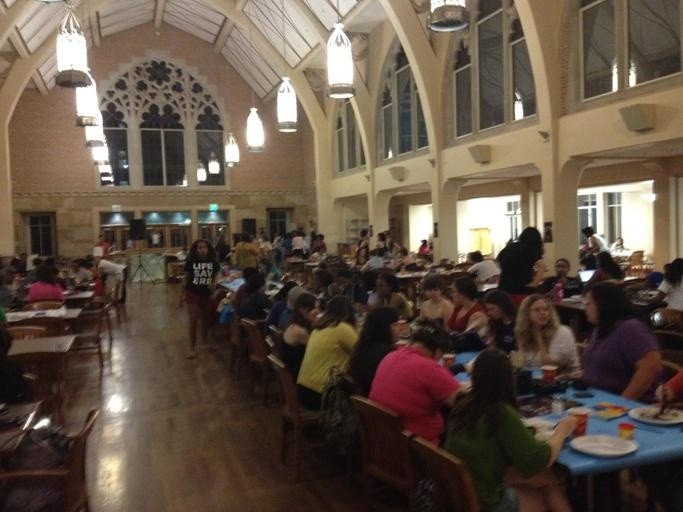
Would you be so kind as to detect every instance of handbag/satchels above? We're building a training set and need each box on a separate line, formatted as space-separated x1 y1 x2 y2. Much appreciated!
409 426 443 511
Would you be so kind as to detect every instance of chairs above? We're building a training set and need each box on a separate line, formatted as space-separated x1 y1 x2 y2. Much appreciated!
0 257 125 512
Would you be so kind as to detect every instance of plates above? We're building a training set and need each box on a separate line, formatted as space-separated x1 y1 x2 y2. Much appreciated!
628 405 683 425
570 435 638 457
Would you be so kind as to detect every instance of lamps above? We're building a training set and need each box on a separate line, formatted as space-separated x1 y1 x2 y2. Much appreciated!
55 0 114 187
428 0 469 33
196 0 356 182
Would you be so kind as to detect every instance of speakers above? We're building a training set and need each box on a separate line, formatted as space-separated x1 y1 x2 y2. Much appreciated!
129 218 146 240
242 218 256 238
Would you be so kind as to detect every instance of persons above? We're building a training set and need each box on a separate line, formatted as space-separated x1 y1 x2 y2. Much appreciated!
1 313 11 411
176 223 682 512
0 253 128 308
93 234 111 255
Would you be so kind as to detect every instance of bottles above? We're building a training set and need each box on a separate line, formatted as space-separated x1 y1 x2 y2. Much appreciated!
618 423 634 440
399 265 406 275
554 283 563 304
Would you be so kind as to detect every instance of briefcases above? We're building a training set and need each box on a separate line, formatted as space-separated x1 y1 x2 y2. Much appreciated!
198 283 227 321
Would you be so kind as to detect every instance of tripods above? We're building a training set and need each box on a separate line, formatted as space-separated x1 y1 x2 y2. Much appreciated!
129 241 156 286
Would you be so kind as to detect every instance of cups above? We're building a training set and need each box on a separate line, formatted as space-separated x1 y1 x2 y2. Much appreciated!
540 364 558 382
442 353 456 368
568 408 589 435
397 318 407 331
222 265 230 277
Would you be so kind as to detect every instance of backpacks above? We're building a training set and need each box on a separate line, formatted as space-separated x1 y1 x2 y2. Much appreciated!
0 423 78 512
295 363 363 457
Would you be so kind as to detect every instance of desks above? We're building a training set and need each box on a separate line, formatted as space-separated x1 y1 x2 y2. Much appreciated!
109 248 188 283
